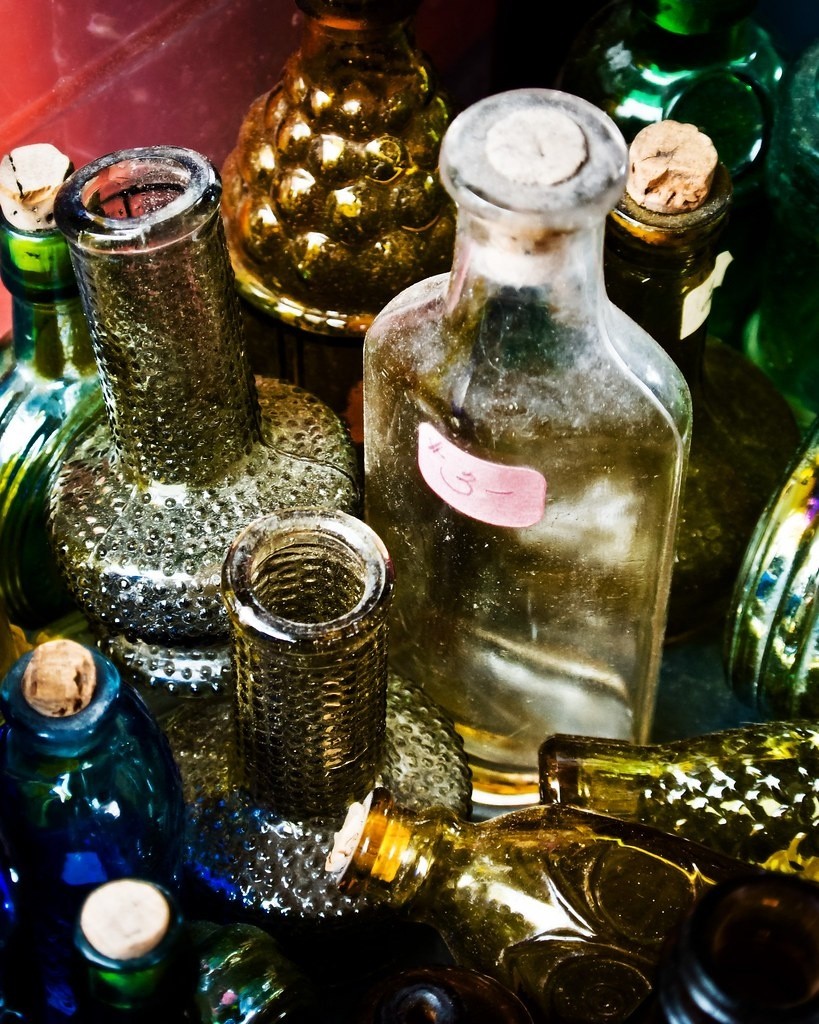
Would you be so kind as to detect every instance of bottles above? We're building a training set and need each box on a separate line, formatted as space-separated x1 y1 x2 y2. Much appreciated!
0 0 819 1024
